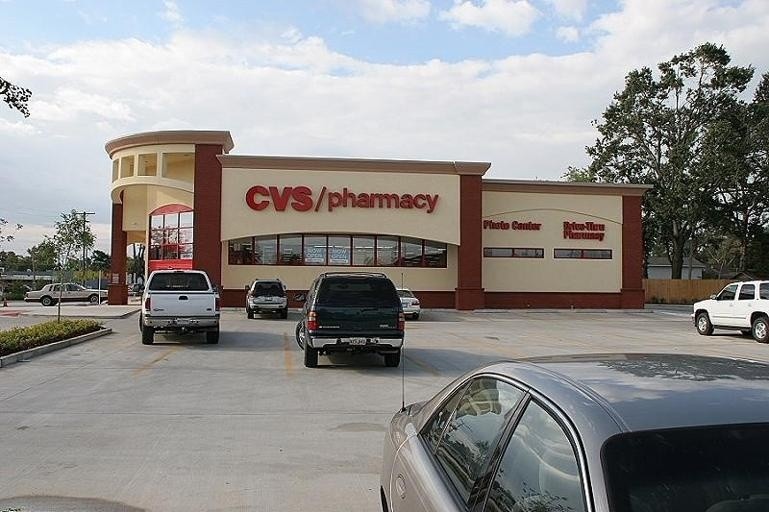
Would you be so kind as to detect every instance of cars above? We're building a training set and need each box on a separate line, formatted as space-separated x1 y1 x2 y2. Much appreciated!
25 282 109 306
378 353 768 510
397 289 420 319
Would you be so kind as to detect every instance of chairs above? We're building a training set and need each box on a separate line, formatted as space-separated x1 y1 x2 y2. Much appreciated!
270 284 278 290
191 279 200 287
158 279 168 287
256 285 263 289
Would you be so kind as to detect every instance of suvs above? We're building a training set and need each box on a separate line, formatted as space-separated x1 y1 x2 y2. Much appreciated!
691 281 769 343
296 270 404 368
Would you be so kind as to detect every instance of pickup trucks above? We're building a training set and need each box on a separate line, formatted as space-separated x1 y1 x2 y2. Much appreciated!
245 278 287 318
133 269 219 344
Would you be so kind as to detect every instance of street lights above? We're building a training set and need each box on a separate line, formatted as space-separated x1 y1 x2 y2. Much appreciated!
76 211 93 273
